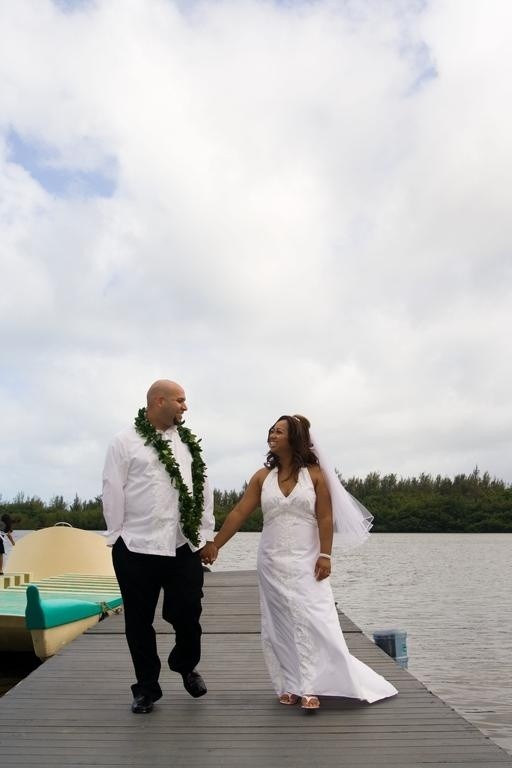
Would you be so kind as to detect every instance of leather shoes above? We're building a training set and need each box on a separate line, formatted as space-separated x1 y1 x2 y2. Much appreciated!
181 667 208 699
130 682 163 714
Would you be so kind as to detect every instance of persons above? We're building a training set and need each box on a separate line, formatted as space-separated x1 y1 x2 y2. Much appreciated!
0 514 15 575
202 415 398 709
95 379 218 713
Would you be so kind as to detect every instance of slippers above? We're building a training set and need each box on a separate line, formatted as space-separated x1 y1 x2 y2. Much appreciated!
301 695 321 711
279 690 298 706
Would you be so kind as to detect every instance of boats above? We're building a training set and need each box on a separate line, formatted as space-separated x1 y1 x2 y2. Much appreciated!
1 518 123 662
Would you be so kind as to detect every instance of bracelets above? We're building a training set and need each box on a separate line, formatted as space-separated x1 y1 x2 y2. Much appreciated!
319 553 331 560
